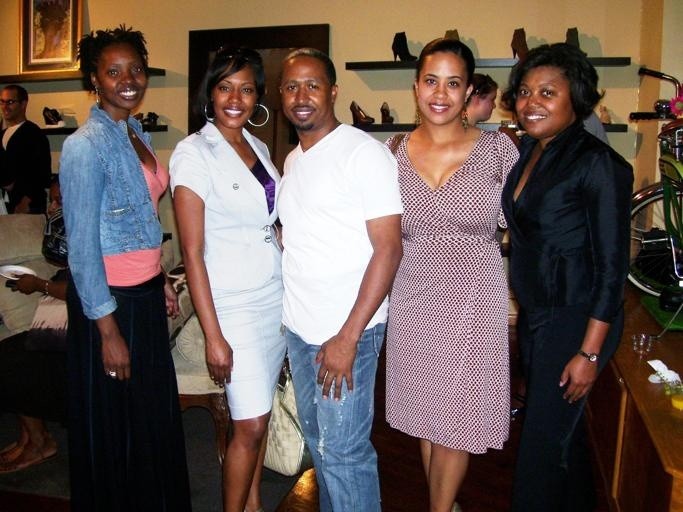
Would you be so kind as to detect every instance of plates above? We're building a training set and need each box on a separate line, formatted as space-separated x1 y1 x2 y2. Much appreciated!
0 264 36 282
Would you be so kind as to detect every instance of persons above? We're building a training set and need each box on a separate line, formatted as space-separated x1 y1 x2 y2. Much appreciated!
495 99 613 148
1 176 89 474
276 48 404 511
462 72 498 128
498 43 634 510
58 25 193 511
168 42 284 511
383 39 521 512
1 84 52 216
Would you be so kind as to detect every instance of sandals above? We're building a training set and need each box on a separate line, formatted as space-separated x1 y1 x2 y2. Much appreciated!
0 435 62 474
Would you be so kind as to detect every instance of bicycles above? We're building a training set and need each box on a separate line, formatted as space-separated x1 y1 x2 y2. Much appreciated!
617 67 683 327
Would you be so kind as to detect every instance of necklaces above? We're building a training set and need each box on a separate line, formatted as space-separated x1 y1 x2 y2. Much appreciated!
128 130 137 140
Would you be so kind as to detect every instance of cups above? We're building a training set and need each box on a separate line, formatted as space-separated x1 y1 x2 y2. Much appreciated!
630 333 651 357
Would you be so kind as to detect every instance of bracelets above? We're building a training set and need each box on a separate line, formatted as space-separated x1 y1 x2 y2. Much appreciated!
578 350 600 362
45 279 48 296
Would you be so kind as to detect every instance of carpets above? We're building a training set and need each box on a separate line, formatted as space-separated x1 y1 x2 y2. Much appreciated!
0 409 312 512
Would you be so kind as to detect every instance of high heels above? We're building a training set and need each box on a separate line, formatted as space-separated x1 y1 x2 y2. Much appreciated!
132 111 160 126
510 29 528 59
43 107 62 125
380 101 394 124
564 27 588 57
392 31 418 61
350 100 375 124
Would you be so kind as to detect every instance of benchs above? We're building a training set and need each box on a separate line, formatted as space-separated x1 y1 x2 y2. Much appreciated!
160 260 231 471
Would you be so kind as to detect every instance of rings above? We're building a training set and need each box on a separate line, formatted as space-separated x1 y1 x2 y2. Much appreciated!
108 370 116 377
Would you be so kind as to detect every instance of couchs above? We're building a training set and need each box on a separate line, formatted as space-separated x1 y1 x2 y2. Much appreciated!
1 212 194 341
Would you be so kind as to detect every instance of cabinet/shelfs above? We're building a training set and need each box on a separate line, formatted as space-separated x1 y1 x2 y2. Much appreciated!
0 66 172 243
585 285 683 512
345 58 631 132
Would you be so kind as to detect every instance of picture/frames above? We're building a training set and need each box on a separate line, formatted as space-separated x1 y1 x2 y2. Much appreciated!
17 0 83 74
